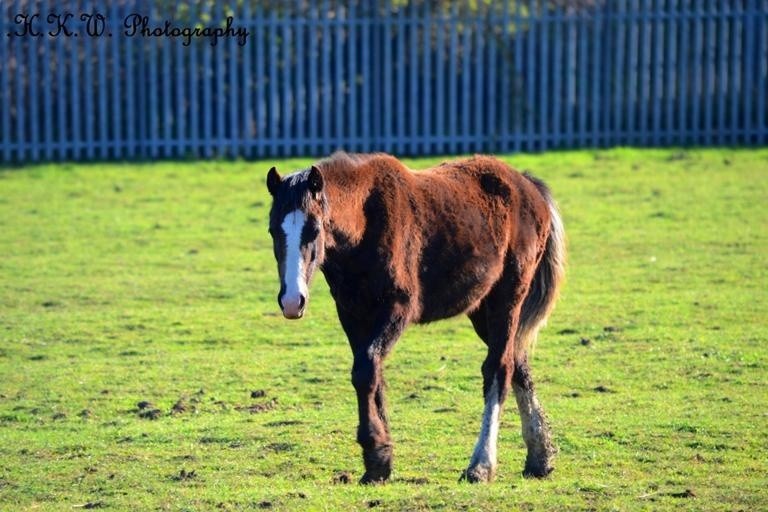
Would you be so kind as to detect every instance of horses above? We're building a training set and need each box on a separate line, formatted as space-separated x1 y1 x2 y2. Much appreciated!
266 149 572 487
